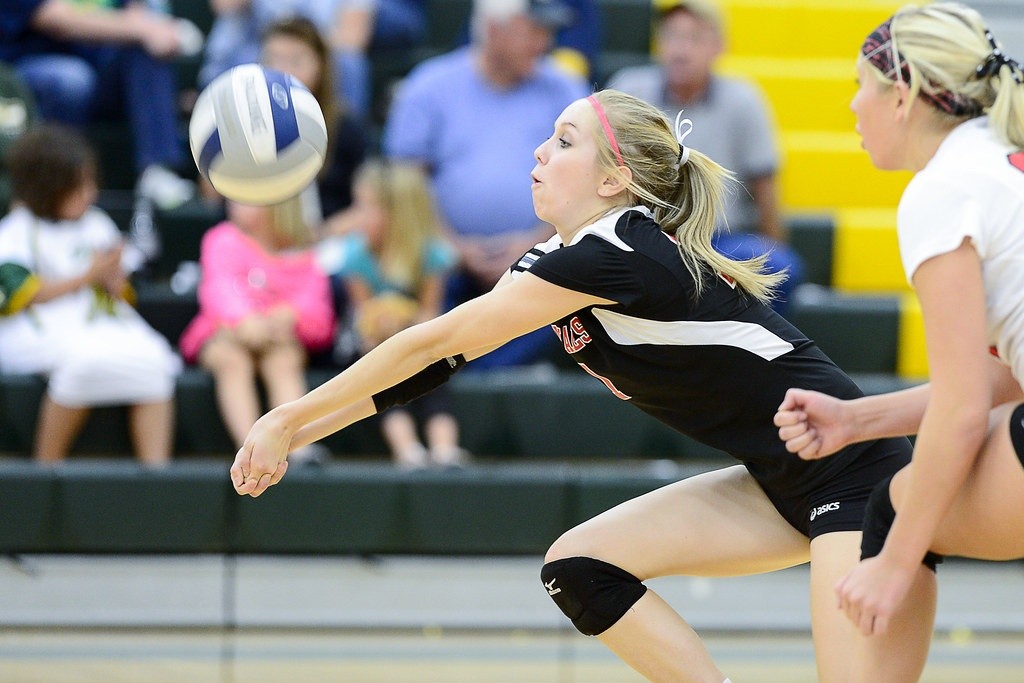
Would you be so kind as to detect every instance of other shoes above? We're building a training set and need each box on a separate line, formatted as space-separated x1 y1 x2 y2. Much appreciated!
141 163 194 211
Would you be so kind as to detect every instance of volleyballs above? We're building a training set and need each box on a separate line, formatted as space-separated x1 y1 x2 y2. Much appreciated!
188 64 328 205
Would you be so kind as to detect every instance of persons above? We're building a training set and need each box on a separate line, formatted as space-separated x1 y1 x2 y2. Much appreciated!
773 2 1024 683
230 90 938 683
0 0 806 466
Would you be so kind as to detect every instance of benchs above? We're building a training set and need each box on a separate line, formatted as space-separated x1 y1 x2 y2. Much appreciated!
3 0 924 563
